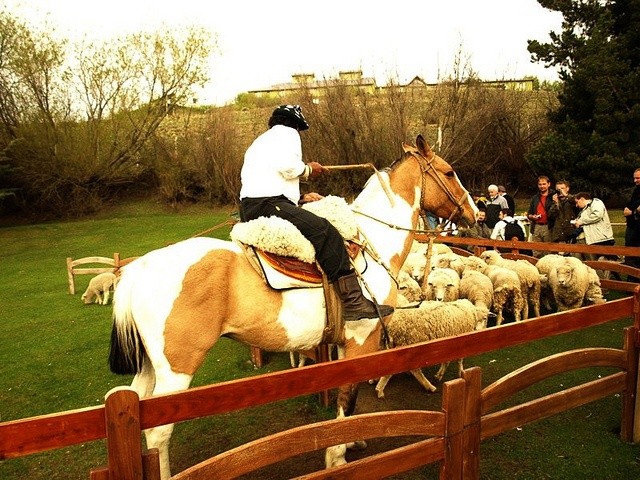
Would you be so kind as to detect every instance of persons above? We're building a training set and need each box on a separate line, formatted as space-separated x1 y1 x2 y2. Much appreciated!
490 209 526 241
469 211 494 239
486 184 509 209
498 185 516 214
424 213 439 231
571 191 616 282
623 168 640 283
471 187 490 212
548 180 579 244
238 104 395 323
526 176 551 258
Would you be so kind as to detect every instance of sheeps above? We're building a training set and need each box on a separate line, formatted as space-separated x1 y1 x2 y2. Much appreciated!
81 271 118 305
288 239 608 399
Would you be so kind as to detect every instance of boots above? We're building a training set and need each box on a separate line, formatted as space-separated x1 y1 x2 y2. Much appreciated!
332 267 394 321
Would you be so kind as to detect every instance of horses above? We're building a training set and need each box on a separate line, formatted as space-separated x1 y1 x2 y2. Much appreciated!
107 133 481 480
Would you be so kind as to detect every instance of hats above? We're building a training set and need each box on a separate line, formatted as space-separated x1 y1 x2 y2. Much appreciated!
273 104 310 129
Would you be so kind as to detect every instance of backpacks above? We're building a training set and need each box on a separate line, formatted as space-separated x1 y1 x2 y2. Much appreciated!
499 220 524 241
